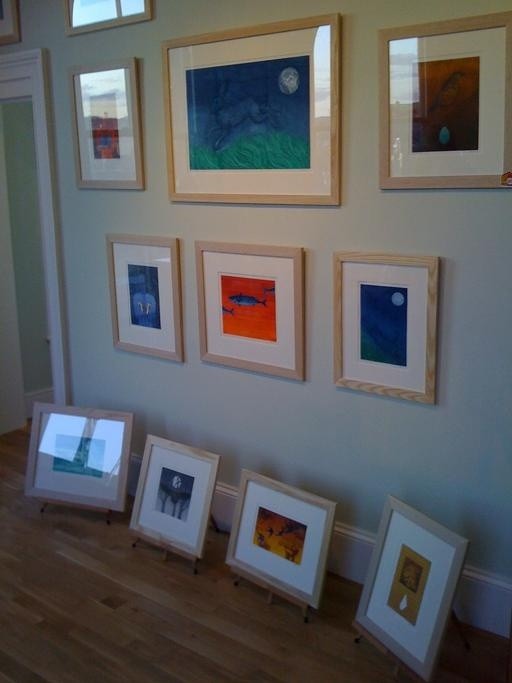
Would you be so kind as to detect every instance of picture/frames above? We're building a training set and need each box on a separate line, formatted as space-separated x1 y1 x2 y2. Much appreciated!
127 432 221 560
353 493 471 682
22 398 136 512
65 0 153 36
161 13 344 205
69 57 146 189
0 0 22 46
105 233 185 363
192 238 307 382
376 10 511 189
332 250 438 405
224 467 338 609
